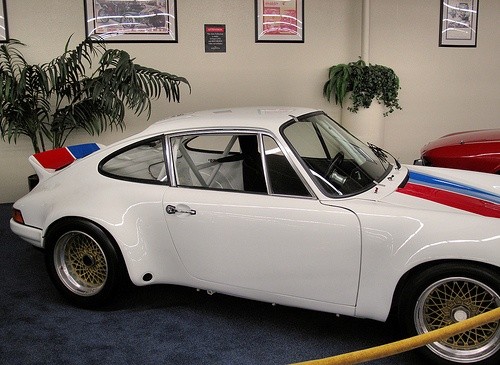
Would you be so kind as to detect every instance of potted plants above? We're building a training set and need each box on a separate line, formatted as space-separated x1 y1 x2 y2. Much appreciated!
321 56 401 152
0 36 193 190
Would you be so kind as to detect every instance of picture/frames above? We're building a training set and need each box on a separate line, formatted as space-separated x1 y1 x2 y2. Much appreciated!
439 0 480 48
255 0 305 43
84 0 177 43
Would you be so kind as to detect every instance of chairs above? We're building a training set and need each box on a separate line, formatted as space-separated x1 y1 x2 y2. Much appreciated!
242 147 309 195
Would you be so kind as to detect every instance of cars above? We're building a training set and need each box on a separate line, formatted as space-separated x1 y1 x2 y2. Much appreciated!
414 128 500 176
94 0 166 28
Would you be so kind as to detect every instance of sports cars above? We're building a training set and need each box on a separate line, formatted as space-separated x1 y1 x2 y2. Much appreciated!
9 106 500 365
